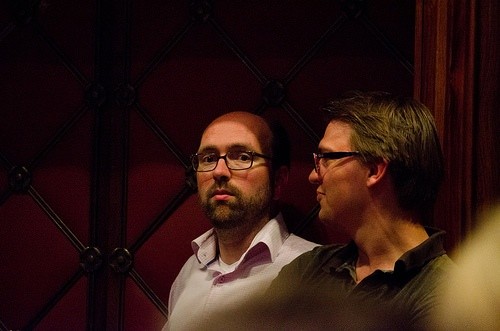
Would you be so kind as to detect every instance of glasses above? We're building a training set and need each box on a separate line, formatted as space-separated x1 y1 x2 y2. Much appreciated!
312 151 361 173
190 150 271 172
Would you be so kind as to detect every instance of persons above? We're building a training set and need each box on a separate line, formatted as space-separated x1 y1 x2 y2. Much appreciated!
255 91 463 331
162 108 327 331
